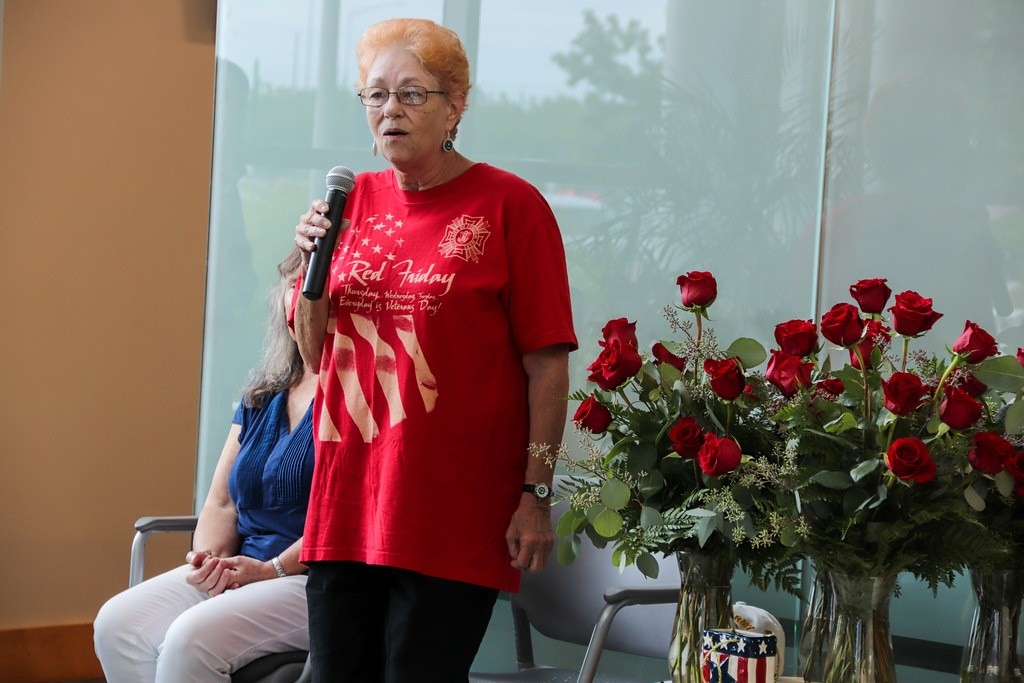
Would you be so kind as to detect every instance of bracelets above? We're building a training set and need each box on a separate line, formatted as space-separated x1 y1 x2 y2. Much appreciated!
271 556 287 577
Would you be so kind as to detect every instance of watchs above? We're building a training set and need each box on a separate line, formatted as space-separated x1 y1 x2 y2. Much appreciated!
522 483 555 502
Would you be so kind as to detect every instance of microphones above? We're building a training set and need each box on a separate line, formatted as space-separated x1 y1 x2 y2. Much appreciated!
302 166 357 301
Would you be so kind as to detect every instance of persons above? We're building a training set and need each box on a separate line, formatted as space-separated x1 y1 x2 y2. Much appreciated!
92 248 319 683
288 17 580 683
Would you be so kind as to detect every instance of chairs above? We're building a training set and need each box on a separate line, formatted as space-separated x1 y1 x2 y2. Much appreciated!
127 476 685 683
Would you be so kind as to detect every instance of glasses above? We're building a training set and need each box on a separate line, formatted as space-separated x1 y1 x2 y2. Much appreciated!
357 85 448 107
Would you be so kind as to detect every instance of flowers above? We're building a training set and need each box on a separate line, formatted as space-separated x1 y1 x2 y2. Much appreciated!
522 266 1024 606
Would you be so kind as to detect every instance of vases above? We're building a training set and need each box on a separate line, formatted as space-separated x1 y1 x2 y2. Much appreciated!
959 564 1024 683
668 549 731 683
797 569 896 683
